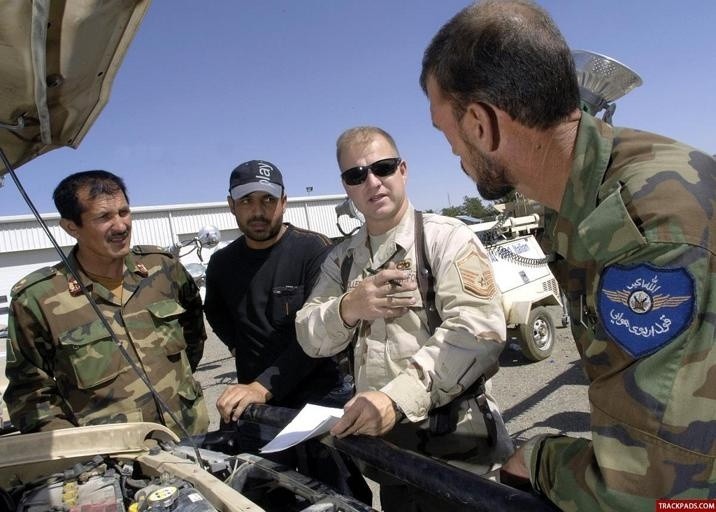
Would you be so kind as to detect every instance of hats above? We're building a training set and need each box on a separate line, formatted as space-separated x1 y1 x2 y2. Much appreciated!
229 160 283 201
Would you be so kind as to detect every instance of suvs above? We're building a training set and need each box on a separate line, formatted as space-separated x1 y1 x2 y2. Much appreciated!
469 213 566 361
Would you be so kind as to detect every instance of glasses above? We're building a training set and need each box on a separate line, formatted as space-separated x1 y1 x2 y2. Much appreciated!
341 158 401 184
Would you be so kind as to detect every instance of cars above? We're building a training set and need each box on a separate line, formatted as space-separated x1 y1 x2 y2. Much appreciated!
0 1 558 511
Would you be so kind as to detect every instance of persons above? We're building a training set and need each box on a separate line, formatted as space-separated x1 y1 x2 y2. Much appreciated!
294 125 516 511
419 1 715 512
204 158 373 510
2 170 211 440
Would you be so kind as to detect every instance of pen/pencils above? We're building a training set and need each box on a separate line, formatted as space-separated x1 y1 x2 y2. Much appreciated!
366 267 402 286
